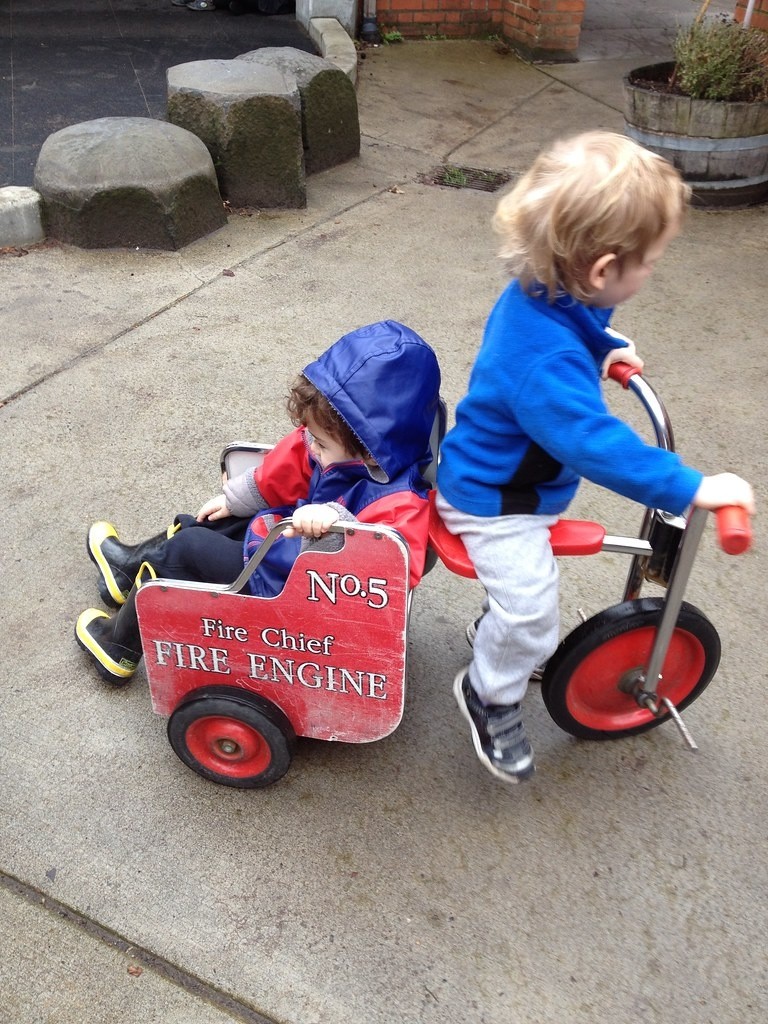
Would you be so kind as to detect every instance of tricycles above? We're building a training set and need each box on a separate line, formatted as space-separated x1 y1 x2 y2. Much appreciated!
135 362 753 792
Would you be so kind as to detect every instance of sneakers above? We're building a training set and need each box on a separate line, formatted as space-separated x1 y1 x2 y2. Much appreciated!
452 667 536 783
466 614 546 680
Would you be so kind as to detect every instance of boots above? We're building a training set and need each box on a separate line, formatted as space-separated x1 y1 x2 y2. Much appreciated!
87 521 178 608
75 563 158 685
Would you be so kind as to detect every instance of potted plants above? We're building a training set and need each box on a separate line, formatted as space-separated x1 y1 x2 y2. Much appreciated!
621 10 768 181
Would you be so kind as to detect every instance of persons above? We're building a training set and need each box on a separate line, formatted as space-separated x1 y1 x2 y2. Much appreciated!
436 131 756 788
74 316 440 689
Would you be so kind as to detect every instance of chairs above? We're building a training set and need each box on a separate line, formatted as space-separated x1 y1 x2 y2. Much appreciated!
135 395 447 742
